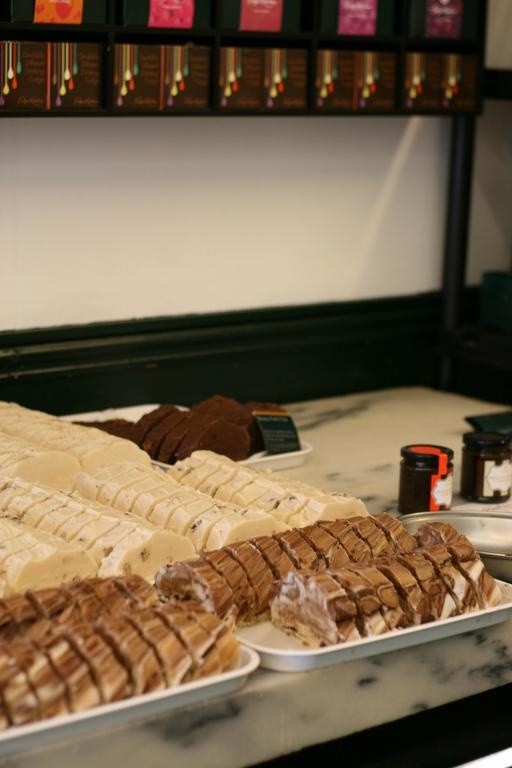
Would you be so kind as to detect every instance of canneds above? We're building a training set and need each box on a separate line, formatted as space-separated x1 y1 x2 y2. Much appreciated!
400 444 453 515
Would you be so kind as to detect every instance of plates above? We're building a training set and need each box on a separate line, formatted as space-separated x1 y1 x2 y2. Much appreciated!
54 398 310 481
218 579 512 673
0 633 258 749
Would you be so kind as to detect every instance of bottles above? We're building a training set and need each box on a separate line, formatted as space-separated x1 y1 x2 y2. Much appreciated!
394 445 455 513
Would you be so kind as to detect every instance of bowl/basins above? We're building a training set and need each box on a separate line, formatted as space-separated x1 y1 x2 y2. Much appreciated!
392 505 512 578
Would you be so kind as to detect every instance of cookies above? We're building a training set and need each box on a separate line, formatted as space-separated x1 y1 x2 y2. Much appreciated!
1 513 503 731
1 395 370 596
